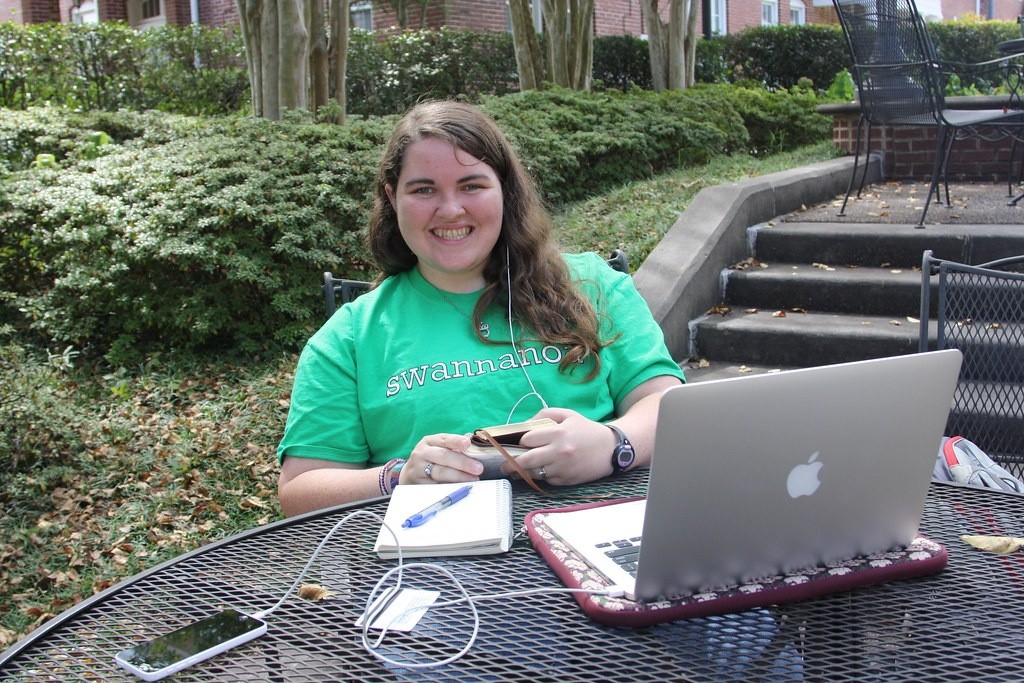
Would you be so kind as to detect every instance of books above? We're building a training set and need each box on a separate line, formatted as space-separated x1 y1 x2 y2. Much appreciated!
372 415 559 557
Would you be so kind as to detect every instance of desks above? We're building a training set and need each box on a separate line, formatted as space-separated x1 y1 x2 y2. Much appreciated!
0 464 1024 683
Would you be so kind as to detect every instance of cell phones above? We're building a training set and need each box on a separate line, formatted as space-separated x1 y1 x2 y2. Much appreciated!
115 609 267 682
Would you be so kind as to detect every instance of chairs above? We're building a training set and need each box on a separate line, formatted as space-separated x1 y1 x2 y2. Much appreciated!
919 250 1024 483
834 0 1024 228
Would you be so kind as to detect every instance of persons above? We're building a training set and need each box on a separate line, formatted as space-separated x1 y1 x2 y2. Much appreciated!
274 95 686 518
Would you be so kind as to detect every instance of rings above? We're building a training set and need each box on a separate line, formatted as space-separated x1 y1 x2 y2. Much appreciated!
424 463 434 479
539 466 546 481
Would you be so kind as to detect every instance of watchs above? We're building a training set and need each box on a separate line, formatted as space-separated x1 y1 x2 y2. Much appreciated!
606 425 636 478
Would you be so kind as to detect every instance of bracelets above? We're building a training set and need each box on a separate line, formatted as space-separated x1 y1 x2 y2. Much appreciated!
378 458 407 497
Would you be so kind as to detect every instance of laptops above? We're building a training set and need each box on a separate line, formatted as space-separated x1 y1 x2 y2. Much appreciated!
541 351 962 603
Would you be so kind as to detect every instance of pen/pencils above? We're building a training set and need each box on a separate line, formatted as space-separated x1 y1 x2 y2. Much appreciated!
401 485 473 528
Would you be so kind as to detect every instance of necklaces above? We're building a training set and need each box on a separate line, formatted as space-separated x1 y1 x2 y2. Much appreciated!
418 271 512 338
